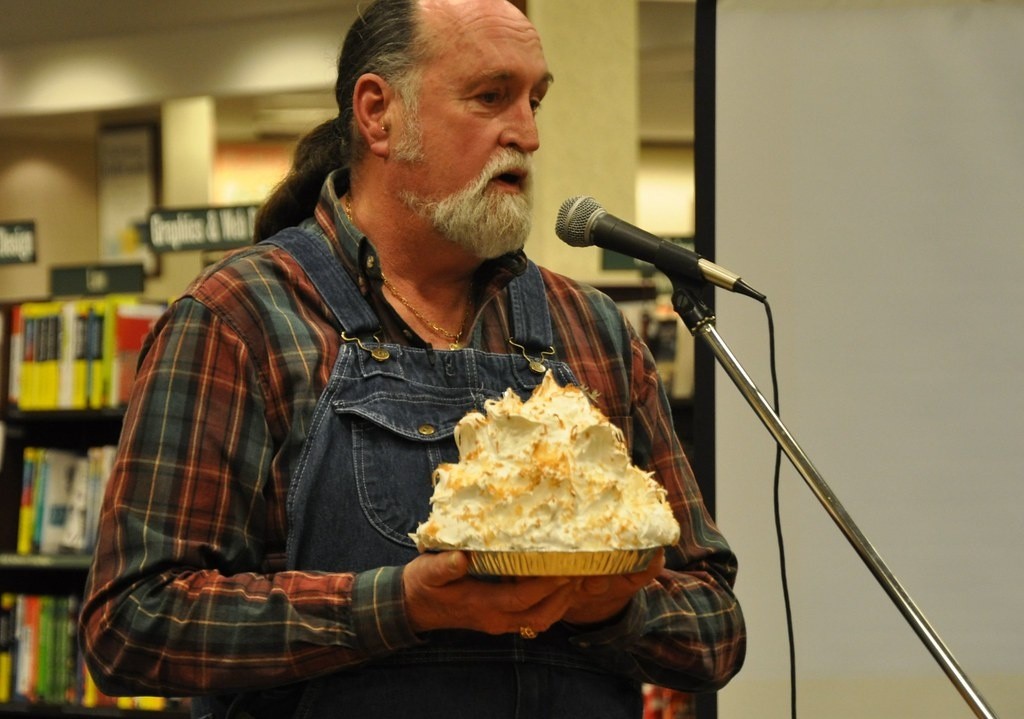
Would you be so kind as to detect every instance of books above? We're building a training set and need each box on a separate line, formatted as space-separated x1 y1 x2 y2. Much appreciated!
8 295 163 409
1 592 173 713
18 447 124 552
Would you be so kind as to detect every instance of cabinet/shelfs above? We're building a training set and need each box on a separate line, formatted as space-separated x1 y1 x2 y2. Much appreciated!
0 287 215 717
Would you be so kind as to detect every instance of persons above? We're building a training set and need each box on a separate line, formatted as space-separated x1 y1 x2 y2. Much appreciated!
77 2 750 719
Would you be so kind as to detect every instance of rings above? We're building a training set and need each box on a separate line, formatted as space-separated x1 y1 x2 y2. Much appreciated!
520 625 535 641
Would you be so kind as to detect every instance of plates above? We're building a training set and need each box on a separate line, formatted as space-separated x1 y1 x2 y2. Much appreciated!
427 545 667 578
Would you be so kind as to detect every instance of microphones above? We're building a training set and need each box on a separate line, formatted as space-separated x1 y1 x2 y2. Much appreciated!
555 196 766 305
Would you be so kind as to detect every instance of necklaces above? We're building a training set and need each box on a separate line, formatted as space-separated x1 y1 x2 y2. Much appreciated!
341 193 478 354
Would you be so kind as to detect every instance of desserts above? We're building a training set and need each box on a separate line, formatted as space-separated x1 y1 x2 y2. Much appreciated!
414 368 680 553
